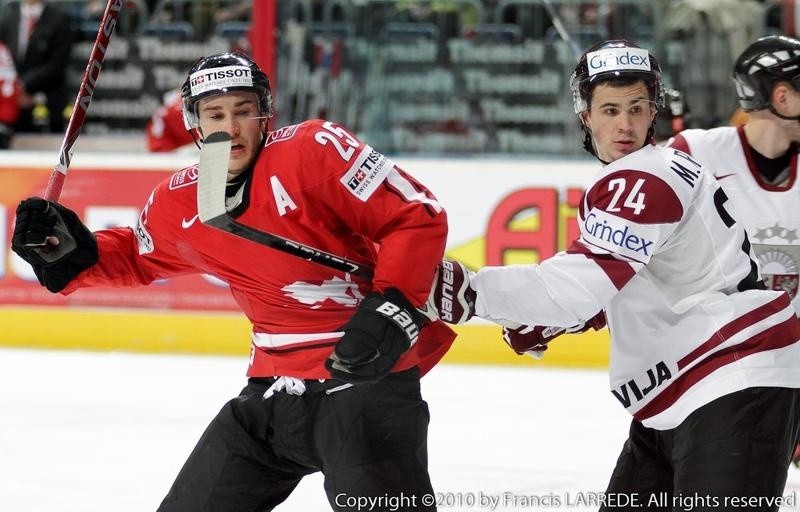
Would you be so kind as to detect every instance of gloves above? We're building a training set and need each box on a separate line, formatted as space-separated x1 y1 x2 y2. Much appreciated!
322 286 429 386
9 195 100 294
416 259 478 327
502 307 607 359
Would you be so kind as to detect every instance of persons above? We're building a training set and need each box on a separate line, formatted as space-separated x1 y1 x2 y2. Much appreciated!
11 50 458 512
416 35 800 512
0 0 73 150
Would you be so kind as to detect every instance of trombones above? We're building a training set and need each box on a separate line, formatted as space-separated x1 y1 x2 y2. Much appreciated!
197 131 374 281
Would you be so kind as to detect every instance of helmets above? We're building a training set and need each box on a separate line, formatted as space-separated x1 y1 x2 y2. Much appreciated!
569 37 664 108
180 49 275 119
730 34 800 114
